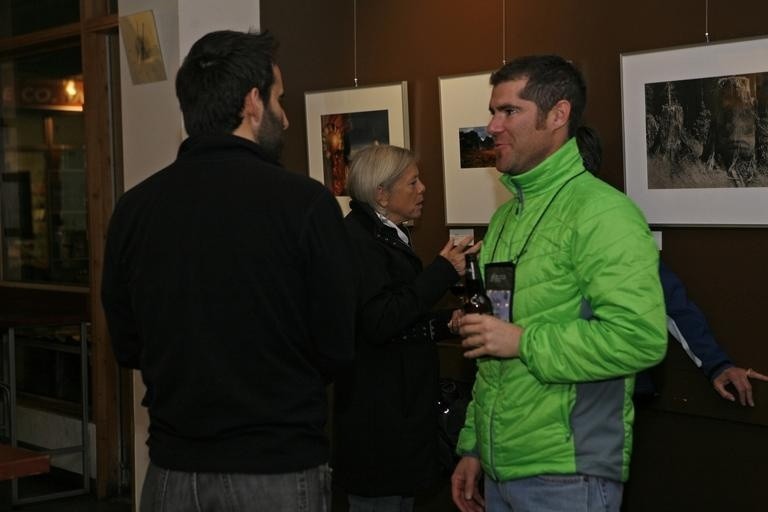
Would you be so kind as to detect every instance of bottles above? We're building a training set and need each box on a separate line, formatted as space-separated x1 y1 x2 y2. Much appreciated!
461 252 494 360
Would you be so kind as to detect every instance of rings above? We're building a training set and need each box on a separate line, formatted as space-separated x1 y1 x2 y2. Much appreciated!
745 367 755 378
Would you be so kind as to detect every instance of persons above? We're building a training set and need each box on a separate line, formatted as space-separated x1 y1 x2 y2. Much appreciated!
332 144 487 510
99 28 355 511
449 52 669 512
573 129 767 408
